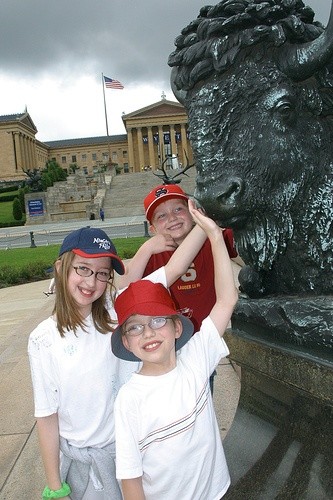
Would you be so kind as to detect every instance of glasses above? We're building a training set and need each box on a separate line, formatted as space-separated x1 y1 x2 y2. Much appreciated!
69 263 114 282
121 317 175 336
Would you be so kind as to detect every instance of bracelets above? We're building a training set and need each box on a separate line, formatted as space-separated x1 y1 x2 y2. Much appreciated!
41 482 72 499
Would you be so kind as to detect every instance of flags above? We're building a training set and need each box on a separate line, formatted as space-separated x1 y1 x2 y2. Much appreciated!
103 76 124 89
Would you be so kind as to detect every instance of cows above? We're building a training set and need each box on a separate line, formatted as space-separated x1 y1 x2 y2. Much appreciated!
166 0 333 298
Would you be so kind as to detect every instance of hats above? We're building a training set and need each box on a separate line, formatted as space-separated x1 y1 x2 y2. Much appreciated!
58 226 125 275
110 279 195 361
144 184 189 221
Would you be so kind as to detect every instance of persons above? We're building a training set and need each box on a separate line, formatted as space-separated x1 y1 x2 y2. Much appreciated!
22 168 36 181
99 208 105 222
29 206 213 500
120 185 238 397
43 260 57 297
179 161 183 169
141 164 152 171
111 199 239 500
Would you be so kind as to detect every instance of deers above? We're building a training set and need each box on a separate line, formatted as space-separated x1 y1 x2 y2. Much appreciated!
153 148 197 185
